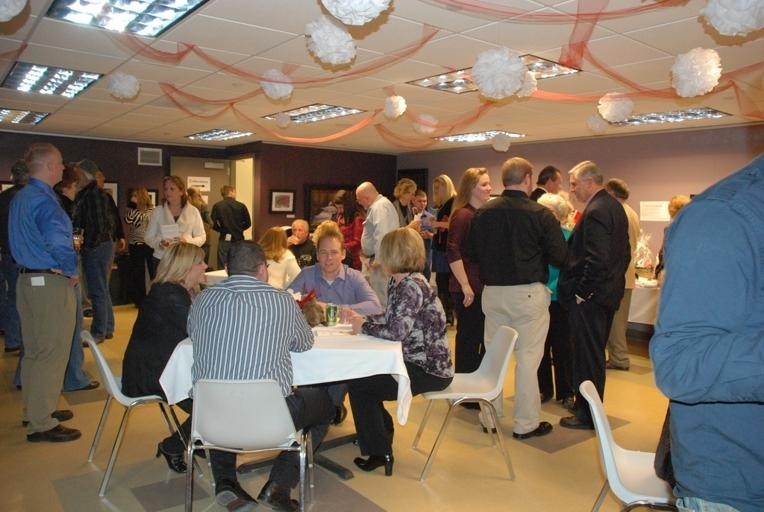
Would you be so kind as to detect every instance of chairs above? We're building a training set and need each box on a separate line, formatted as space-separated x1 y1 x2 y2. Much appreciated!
411 325 519 483
185 379 314 512
80 329 204 497
579 380 679 512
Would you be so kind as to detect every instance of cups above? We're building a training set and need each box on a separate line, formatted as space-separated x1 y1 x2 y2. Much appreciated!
72 227 84 253
339 304 352 325
326 304 337 325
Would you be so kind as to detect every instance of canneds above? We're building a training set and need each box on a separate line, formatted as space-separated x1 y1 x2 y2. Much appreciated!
326 302 337 326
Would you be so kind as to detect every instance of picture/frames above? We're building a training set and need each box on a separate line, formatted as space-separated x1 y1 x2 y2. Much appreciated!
268 189 297 215
102 181 119 209
303 183 360 233
146 188 160 207
0 181 17 193
136 145 163 168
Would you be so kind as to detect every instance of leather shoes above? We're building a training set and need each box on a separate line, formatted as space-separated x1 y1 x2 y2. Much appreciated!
256 479 300 512
567 405 577 415
559 416 595 430
22 409 73 427
605 360 629 370
480 423 497 433
27 424 82 443
512 421 553 440
75 381 100 390
329 410 337 424
82 337 105 347
334 396 348 425
215 479 259 512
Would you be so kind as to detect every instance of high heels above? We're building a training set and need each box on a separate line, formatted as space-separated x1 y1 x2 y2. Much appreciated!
155 440 195 474
562 396 574 408
354 452 394 477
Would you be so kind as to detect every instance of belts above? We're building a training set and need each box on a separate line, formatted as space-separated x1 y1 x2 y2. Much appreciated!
16 267 71 279
366 253 375 258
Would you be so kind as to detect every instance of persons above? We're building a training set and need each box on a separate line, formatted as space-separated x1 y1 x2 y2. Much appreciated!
331 175 459 328
555 161 640 428
121 241 207 472
258 220 353 291
653 195 692 287
126 174 251 308
446 168 491 408
285 232 381 424
468 158 576 438
188 242 334 512
0 142 125 443
347 228 455 476
648 153 764 512
